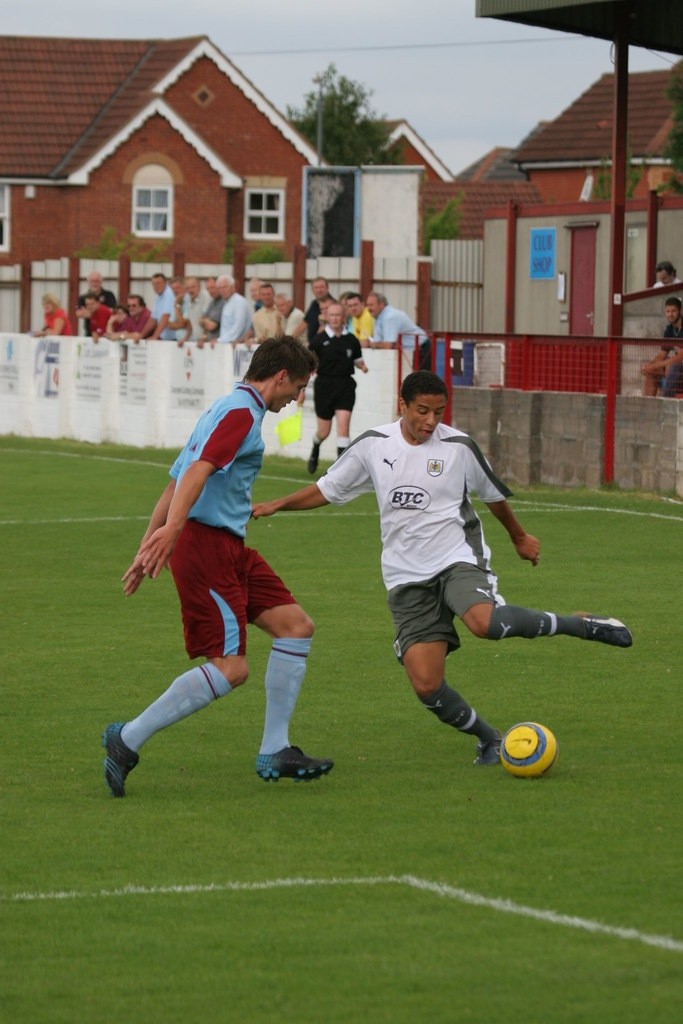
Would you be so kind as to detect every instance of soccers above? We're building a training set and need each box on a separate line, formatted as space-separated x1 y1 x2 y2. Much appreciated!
499 722 558 778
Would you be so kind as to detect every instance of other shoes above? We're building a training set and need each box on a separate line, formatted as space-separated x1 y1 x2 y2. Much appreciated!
308 447 318 473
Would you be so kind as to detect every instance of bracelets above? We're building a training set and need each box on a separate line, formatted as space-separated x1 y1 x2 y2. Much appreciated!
175 304 180 308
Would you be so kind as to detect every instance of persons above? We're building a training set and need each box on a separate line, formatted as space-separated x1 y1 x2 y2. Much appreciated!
102 335 334 799
75 271 212 347
653 261 681 288
249 371 633 765
640 297 683 398
359 292 432 370
197 275 308 349
299 305 369 474
292 279 374 338
33 294 71 337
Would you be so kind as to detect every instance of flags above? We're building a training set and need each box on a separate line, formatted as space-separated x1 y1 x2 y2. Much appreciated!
275 412 302 446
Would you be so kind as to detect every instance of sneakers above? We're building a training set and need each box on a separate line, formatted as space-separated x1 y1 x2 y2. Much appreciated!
571 609 633 648
100 723 139 798
256 746 335 782
473 728 503 765
